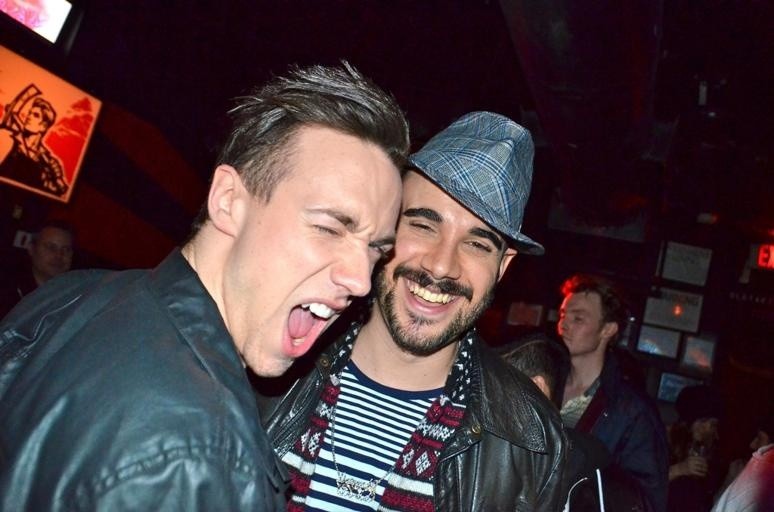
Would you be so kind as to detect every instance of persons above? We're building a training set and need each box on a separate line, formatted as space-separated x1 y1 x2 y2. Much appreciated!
247 110 569 512
3 65 409 512
3 218 73 318
504 274 773 511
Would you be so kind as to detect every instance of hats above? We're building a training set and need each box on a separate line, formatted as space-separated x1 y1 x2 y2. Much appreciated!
409 111 545 256
